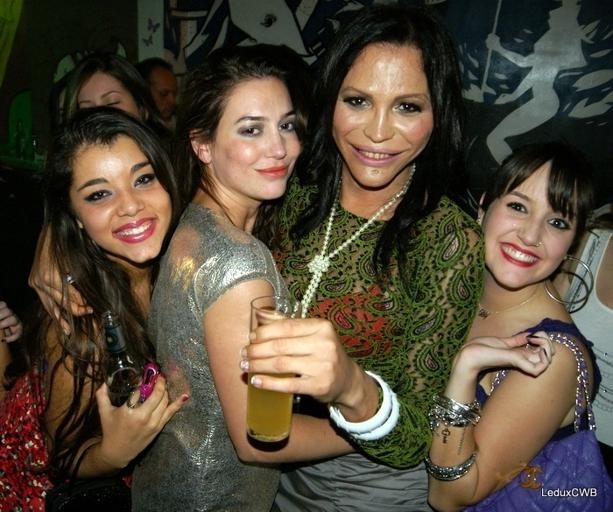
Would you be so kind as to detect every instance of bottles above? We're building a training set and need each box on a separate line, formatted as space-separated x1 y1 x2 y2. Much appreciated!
102 309 145 409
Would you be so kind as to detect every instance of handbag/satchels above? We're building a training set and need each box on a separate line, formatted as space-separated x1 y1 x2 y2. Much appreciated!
464 330 613 512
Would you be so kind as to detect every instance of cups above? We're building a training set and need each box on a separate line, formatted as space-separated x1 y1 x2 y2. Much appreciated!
244 295 301 445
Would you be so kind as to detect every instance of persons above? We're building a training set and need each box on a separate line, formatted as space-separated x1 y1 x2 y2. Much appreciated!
1 0 613 512
